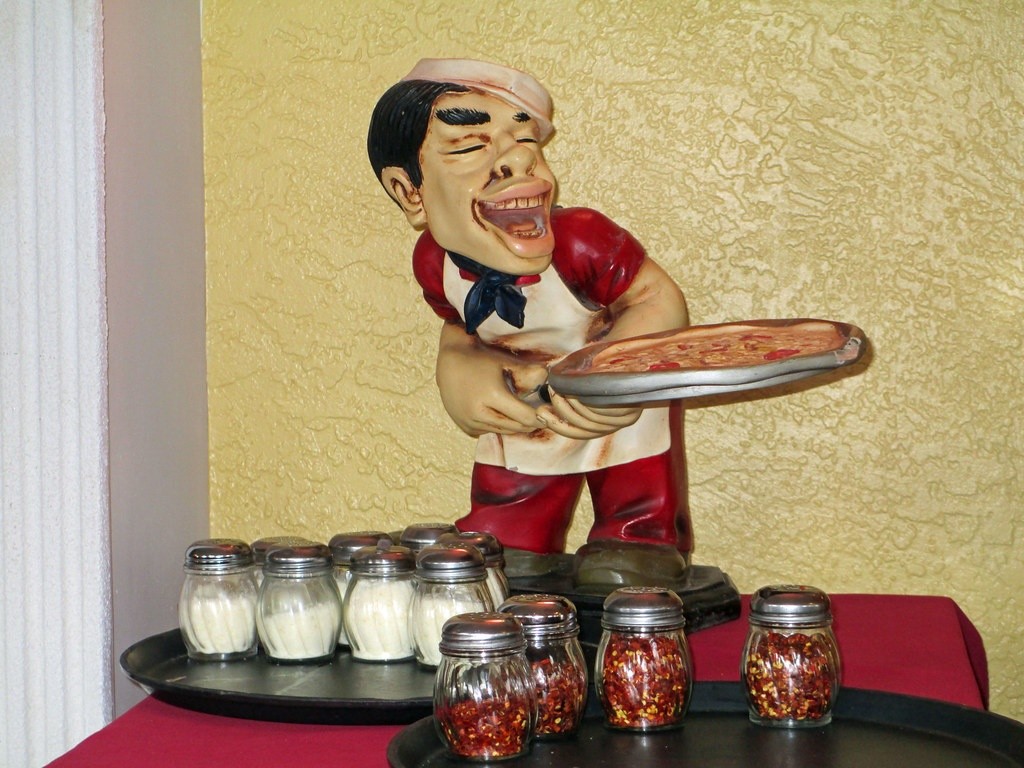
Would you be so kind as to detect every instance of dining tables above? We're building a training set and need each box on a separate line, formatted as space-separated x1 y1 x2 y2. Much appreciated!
43 593 991 768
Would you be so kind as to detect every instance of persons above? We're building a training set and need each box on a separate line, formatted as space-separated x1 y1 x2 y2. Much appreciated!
366 58 695 587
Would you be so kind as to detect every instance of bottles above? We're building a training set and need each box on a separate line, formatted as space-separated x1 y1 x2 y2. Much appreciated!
250 535 313 587
399 523 460 554
406 543 495 673
497 594 588 740
433 612 539 763
177 538 262 661
455 531 510 614
255 546 344 665
343 545 419 664
594 586 692 731
740 584 841 729
327 531 396 653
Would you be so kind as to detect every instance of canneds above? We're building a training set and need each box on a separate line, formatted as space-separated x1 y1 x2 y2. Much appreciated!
177 524 510 670
743 583 840 728
432 587 696 761
